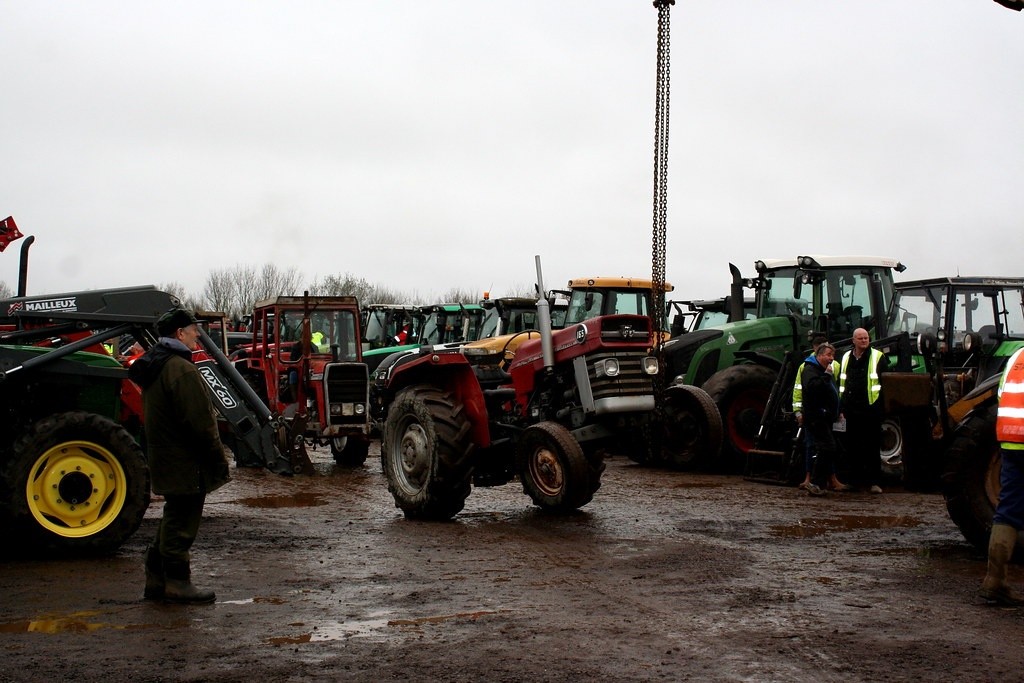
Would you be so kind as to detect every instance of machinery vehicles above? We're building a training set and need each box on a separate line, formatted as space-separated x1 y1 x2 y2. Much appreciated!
0 254 1024 567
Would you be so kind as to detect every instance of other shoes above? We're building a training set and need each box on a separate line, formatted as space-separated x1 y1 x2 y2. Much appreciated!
801 481 815 490
831 481 850 491
145 575 216 605
871 485 884 494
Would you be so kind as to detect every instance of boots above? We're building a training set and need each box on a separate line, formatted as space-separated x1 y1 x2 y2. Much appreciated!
978 523 1024 605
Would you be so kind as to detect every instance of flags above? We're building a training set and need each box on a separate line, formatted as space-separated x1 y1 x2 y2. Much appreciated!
0 216 24 253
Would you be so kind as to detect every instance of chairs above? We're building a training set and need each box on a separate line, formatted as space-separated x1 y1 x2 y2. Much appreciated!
978 323 999 346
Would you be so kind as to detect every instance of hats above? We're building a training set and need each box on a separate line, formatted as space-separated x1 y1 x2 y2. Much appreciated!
156 308 206 337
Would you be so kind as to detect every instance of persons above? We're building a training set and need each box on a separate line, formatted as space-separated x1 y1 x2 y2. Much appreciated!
126 308 233 604
803 341 849 497
289 328 320 362
836 328 891 495
392 313 412 346
278 317 286 342
790 338 841 485
103 338 114 357
974 341 1024 609
